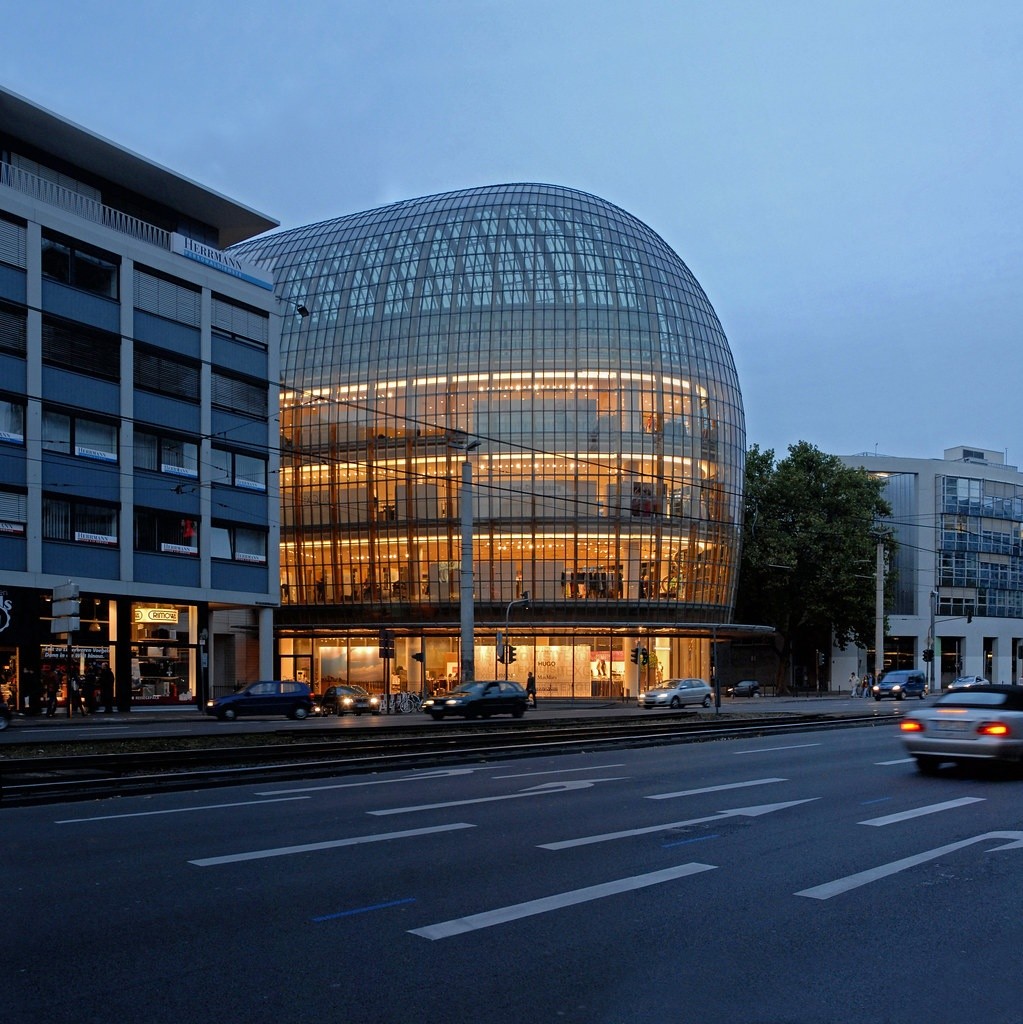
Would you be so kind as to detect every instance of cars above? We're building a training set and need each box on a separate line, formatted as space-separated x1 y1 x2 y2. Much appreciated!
727 680 761 698
948 675 990 691
204 680 317 721
422 680 530 721
899 683 1023 773
321 685 380 717
637 677 715 709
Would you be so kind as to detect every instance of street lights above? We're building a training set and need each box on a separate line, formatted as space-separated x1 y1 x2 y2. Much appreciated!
447 440 482 679
871 530 894 684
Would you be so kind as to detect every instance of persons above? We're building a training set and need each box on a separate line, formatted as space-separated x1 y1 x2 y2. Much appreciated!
98 662 114 713
525 671 538 709
848 672 860 698
316 578 324 602
71 667 96 714
859 673 875 698
46 671 59 717
877 670 885 683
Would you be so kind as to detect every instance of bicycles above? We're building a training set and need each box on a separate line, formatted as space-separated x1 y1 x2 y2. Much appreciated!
377 691 425 714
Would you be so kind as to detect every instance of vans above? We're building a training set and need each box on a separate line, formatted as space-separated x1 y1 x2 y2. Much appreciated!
872 669 928 701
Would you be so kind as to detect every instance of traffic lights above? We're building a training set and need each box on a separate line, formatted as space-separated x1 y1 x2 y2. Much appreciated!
923 650 929 662
498 646 507 664
631 648 638 664
507 645 516 664
642 648 652 665
